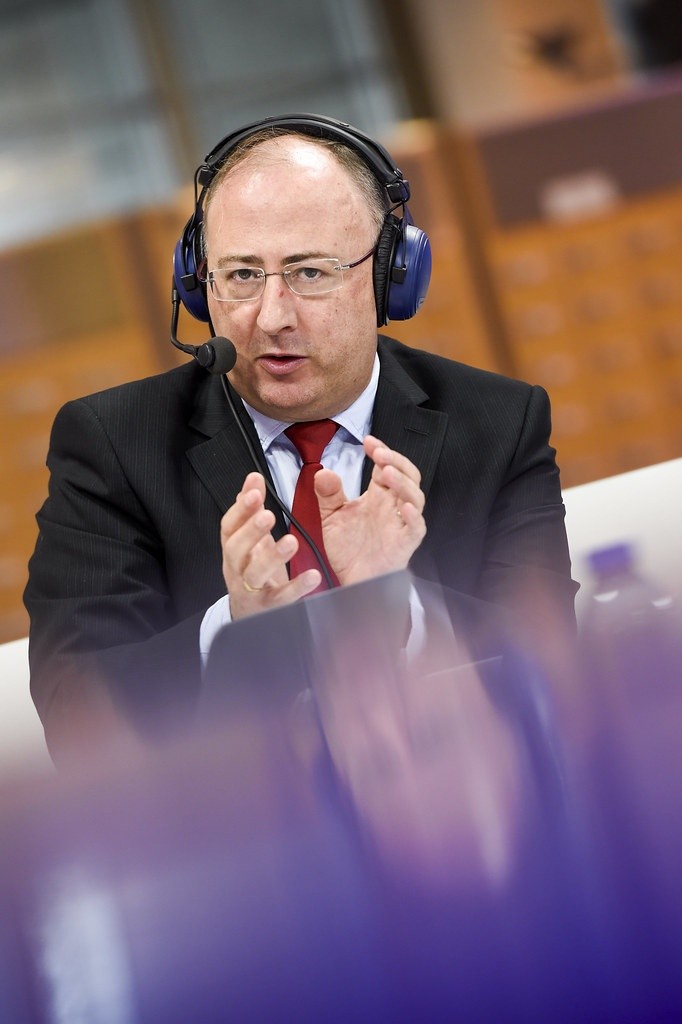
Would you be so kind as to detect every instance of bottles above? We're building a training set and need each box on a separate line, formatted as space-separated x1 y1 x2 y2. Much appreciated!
582 546 664 638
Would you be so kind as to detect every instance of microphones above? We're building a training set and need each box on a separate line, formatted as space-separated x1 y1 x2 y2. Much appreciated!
170 276 237 376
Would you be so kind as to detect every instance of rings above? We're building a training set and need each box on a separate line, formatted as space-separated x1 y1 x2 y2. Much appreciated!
242 579 262 592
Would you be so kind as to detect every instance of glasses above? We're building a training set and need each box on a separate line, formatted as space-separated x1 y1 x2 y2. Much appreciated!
197 241 377 302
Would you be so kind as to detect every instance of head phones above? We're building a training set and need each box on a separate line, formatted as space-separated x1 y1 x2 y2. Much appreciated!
172 113 431 328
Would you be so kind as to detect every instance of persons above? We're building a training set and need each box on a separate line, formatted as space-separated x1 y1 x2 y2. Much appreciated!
21 113 587 785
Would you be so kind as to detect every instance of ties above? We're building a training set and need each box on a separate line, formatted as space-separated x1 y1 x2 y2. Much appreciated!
275 418 342 600
